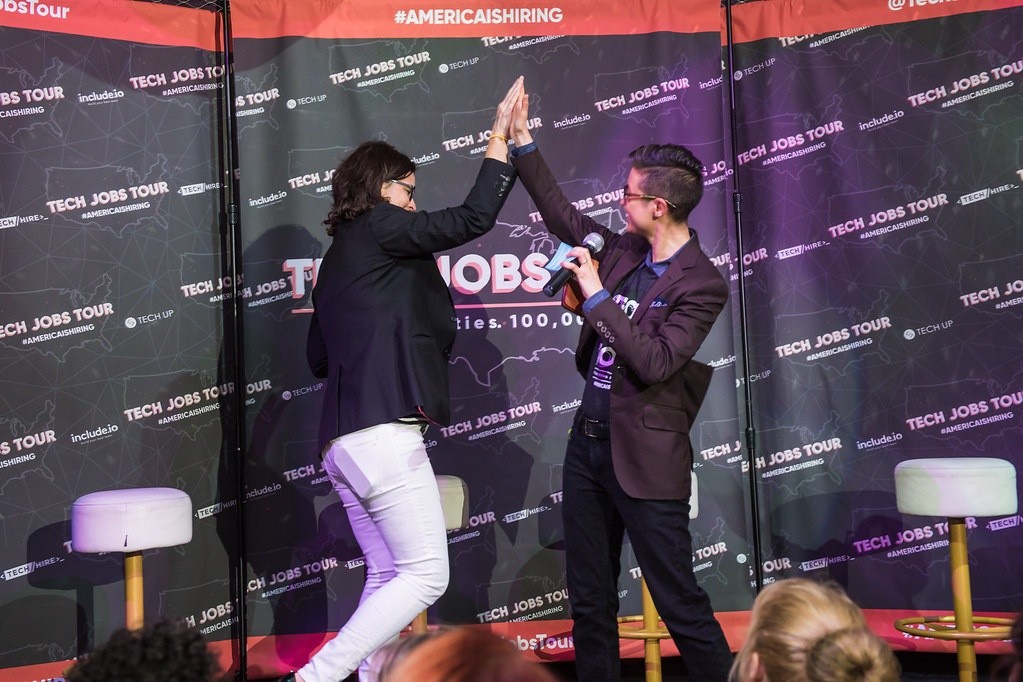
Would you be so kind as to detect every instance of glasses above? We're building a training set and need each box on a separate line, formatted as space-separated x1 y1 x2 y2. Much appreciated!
622 184 676 209
390 179 415 201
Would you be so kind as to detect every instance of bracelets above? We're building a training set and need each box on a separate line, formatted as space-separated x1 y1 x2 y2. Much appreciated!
488 134 508 145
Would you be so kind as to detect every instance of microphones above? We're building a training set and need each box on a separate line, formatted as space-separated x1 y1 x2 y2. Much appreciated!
543 232 604 297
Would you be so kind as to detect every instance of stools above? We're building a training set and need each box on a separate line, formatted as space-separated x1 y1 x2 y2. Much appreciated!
617 471 698 682
895 458 1018 682
412 475 470 635
72 487 193 639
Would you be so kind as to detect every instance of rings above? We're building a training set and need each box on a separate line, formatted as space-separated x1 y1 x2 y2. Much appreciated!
582 259 588 264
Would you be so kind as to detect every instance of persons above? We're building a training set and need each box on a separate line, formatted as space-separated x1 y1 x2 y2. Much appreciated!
509 82 736 682
378 628 552 682
729 578 900 682
1007 611 1023 682
66 618 220 682
277 76 523 682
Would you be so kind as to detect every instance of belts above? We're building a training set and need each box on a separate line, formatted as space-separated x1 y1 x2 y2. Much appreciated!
576 415 610 439
